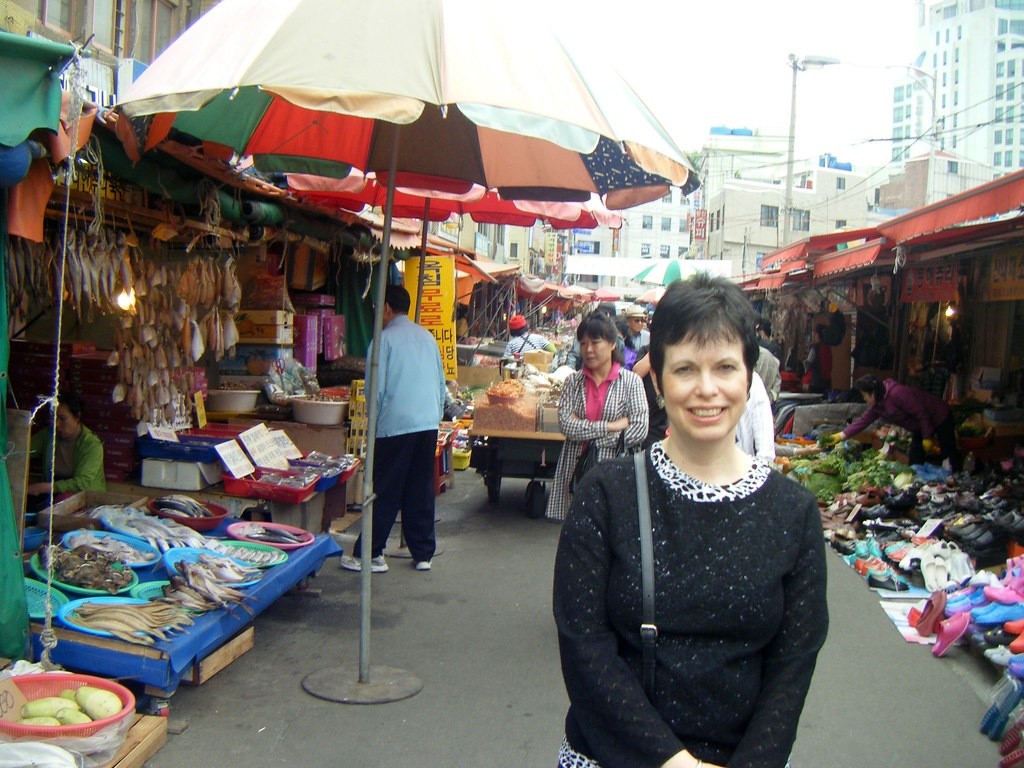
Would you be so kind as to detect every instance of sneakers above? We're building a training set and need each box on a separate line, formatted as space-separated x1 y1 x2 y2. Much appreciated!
413 558 432 570
341 553 388 572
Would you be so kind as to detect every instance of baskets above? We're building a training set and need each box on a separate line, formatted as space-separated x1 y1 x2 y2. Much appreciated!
140 422 360 504
22 509 314 635
0 673 136 740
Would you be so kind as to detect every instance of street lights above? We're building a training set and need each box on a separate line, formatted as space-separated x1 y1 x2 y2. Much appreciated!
907 65 943 207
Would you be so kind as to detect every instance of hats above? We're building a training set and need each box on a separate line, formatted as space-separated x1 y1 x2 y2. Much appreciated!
625 306 647 318
509 315 527 330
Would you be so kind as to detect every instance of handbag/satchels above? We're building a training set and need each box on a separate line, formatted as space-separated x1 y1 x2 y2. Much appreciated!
568 431 625 495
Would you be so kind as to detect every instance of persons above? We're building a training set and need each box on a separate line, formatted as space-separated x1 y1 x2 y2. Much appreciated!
802 324 832 391
825 373 963 474
546 301 786 522
27 391 107 512
456 303 477 345
545 270 833 768
502 314 558 365
341 284 446 572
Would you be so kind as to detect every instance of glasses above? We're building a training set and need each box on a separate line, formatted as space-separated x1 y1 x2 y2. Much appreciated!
629 318 644 322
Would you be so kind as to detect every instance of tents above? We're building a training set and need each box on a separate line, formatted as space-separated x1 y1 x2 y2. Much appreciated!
593 287 620 302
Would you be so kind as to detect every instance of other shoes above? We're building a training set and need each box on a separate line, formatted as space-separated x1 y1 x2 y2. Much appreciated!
814 468 1024 767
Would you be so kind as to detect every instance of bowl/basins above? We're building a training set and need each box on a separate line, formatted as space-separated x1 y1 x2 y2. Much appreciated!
204 388 260 415
147 497 229 531
289 396 349 425
24 528 47 550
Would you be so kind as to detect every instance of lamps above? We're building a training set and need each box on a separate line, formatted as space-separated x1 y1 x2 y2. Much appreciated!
152 203 185 242
820 290 830 307
123 213 139 248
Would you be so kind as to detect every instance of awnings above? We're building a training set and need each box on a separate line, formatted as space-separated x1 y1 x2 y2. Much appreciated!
736 169 1024 295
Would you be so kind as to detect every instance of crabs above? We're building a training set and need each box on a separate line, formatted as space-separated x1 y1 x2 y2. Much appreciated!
42 541 133 593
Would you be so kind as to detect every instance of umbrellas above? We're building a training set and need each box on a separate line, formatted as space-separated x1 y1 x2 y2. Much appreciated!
631 259 699 286
634 286 666 305
271 167 630 324
105 0 702 685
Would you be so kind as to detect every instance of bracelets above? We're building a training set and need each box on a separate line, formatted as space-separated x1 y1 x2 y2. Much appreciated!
696 759 702 768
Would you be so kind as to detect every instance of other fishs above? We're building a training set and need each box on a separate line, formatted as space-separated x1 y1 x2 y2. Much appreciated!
5 224 240 426
148 544 286 621
118 513 205 554
245 526 311 545
65 601 198 645
152 494 213 521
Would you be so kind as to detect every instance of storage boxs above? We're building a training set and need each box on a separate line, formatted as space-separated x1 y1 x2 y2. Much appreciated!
969 365 1024 436
780 372 798 393
524 348 554 374
10 294 475 503
